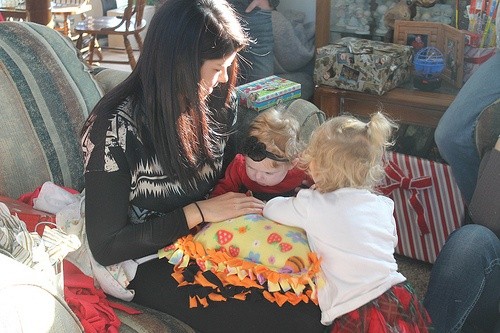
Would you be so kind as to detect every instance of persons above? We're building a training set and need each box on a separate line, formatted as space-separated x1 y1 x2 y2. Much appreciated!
209 107 314 198
422 48 500 333
263 113 432 333
229 0 280 84
80 0 332 333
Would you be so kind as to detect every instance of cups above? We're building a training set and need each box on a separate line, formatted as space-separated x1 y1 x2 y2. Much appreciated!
6 2 16 14
88 17 92 23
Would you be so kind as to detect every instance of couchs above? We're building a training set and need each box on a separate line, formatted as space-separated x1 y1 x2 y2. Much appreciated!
0 20 326 333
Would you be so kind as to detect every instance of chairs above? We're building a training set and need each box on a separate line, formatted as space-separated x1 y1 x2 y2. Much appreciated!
0 0 148 72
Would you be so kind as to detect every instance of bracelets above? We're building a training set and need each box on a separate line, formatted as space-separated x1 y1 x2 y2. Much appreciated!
194 202 205 225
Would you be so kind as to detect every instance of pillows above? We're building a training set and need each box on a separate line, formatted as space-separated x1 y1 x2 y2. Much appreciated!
186 214 319 295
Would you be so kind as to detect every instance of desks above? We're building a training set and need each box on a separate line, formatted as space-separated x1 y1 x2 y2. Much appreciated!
315 79 461 128
0 3 103 61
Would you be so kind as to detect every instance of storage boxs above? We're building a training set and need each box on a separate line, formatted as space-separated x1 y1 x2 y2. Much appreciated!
235 74 301 112
374 151 465 264
316 37 414 97
1 201 57 238
